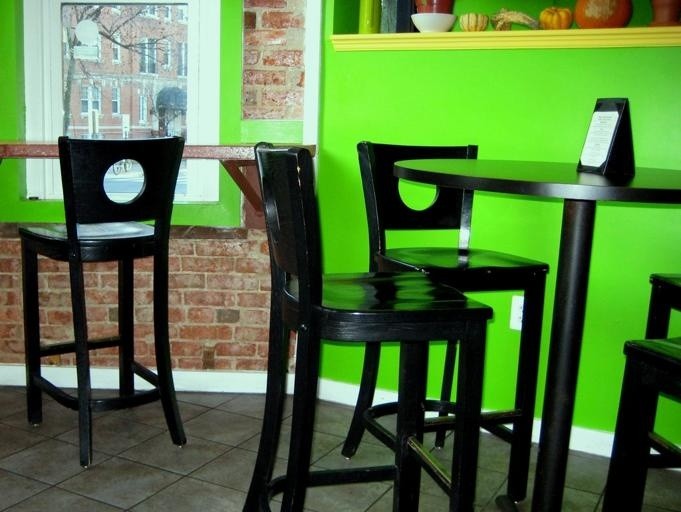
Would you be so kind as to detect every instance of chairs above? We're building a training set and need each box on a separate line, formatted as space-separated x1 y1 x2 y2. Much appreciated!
19 135 187 468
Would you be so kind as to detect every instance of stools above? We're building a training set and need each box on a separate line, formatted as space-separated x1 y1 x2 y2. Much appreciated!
645 270 681 340
241 142 493 509
605 334 681 510
339 141 550 503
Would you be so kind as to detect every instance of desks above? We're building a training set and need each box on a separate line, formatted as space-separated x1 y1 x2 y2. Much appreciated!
392 151 681 508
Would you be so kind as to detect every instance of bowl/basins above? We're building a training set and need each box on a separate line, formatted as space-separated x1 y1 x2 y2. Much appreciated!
411 12 456 32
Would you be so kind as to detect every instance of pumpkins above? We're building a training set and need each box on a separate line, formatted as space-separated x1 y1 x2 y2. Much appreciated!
575 0 633 29
540 7 573 30
459 13 489 32
